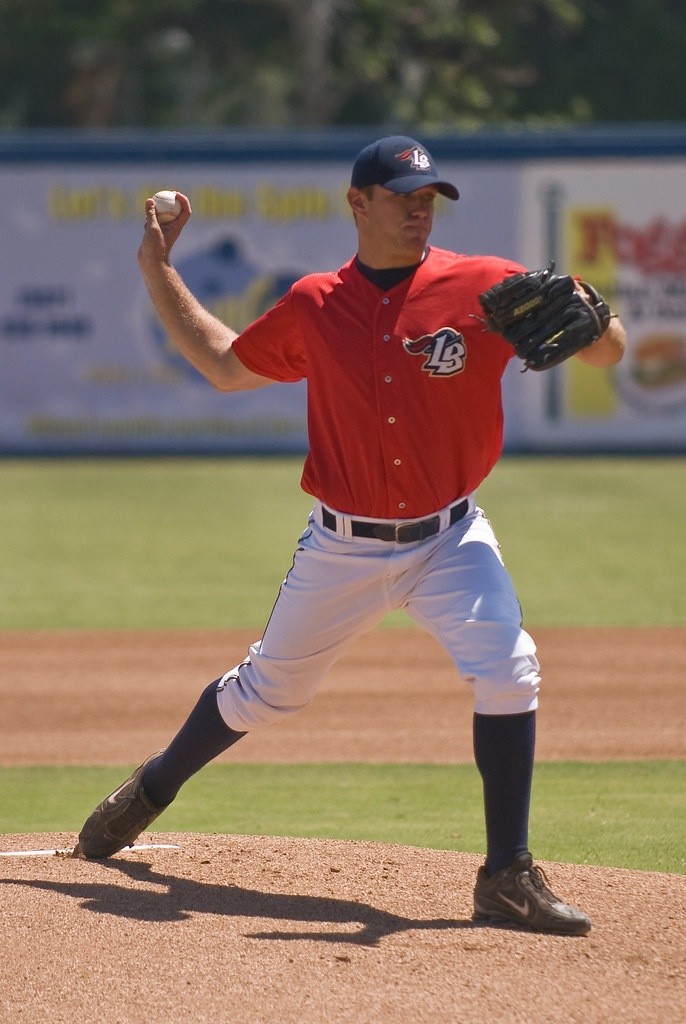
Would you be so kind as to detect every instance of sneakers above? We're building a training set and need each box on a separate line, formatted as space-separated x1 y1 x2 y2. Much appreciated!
473 851 592 936
78 748 184 859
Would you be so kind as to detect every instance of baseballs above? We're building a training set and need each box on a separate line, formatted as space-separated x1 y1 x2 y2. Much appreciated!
152 189 182 225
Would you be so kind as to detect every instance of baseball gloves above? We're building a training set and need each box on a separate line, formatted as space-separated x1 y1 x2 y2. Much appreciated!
477 270 612 372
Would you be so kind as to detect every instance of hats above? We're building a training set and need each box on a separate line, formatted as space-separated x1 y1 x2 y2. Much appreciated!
350 135 461 201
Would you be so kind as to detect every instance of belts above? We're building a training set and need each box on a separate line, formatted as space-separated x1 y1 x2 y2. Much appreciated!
322 497 468 543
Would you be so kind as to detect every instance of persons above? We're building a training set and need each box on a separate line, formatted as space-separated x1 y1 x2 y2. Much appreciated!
81 135 624 935
